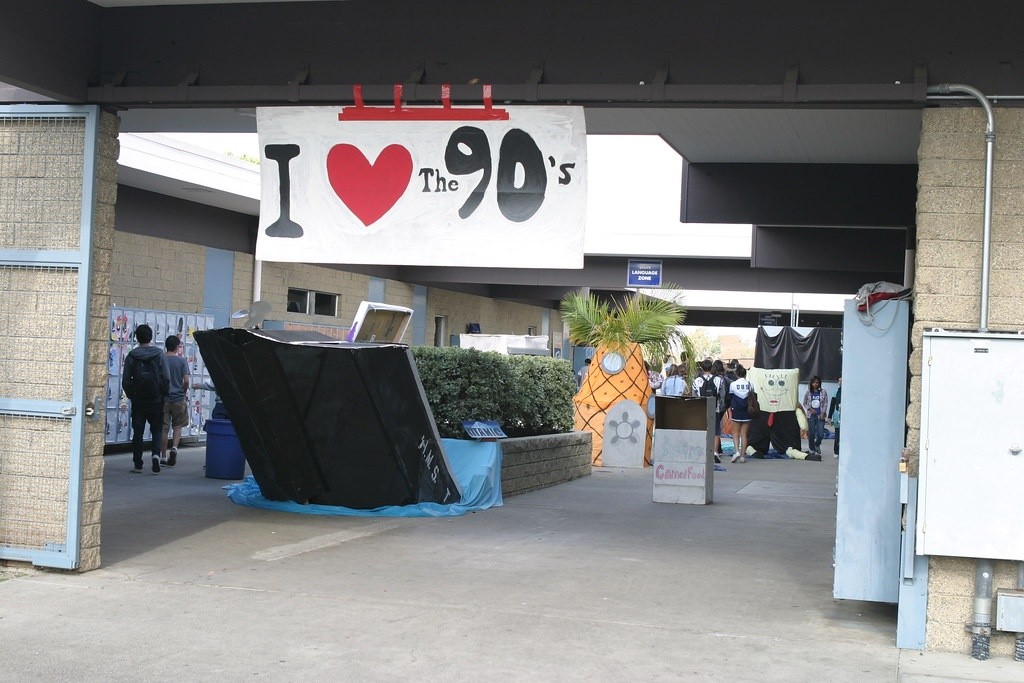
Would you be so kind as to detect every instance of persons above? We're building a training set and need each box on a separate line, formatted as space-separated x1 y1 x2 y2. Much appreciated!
122 324 170 473
828 377 841 458
159 335 190 466
729 364 754 463
803 376 827 461
644 352 738 463
577 359 591 392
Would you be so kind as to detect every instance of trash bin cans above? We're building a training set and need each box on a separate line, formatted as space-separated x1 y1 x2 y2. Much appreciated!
203 419 246 480
653 394 717 505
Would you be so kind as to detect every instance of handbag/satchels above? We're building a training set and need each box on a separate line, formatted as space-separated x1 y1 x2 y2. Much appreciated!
833 404 840 428
747 382 760 418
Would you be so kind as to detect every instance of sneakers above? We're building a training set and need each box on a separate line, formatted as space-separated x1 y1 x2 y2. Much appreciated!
731 452 740 463
740 457 746 463
167 448 177 467
160 456 168 466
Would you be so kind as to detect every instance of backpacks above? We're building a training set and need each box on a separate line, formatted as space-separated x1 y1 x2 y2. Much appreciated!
699 373 718 408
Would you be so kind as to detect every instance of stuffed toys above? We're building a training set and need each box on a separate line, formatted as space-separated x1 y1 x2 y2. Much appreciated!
746 367 821 461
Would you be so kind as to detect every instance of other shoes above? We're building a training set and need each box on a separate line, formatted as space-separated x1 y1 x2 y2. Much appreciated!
834 453 839 458
815 445 821 454
809 449 815 454
151 455 161 473
714 452 721 463
130 468 143 473
